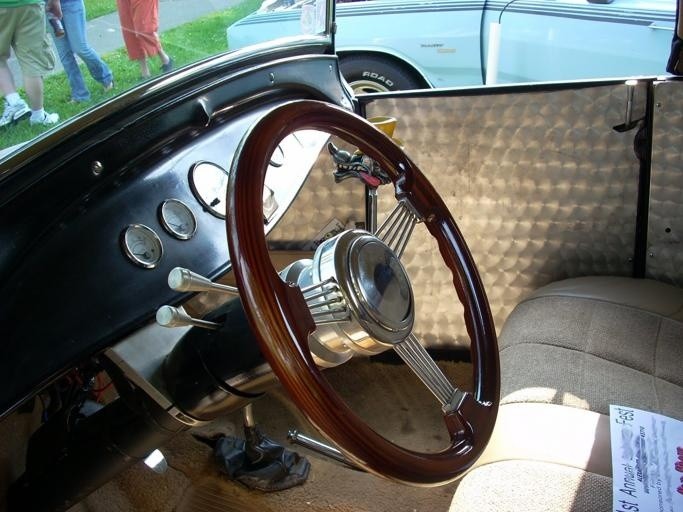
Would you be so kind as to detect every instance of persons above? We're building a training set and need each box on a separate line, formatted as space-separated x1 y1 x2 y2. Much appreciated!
114 0 172 78
44 0 115 104
0 0 66 127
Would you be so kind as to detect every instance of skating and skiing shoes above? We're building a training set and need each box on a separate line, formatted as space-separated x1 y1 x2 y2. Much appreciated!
161 58 173 74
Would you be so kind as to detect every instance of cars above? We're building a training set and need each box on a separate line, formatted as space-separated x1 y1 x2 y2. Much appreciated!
227 1 681 94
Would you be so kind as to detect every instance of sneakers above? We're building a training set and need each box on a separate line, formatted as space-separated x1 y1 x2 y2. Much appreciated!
0 97 30 129
29 109 59 129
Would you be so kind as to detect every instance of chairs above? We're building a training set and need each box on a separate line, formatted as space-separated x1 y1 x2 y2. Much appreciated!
450 274 681 510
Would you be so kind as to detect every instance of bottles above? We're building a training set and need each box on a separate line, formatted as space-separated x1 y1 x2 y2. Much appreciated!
45 9 64 37
